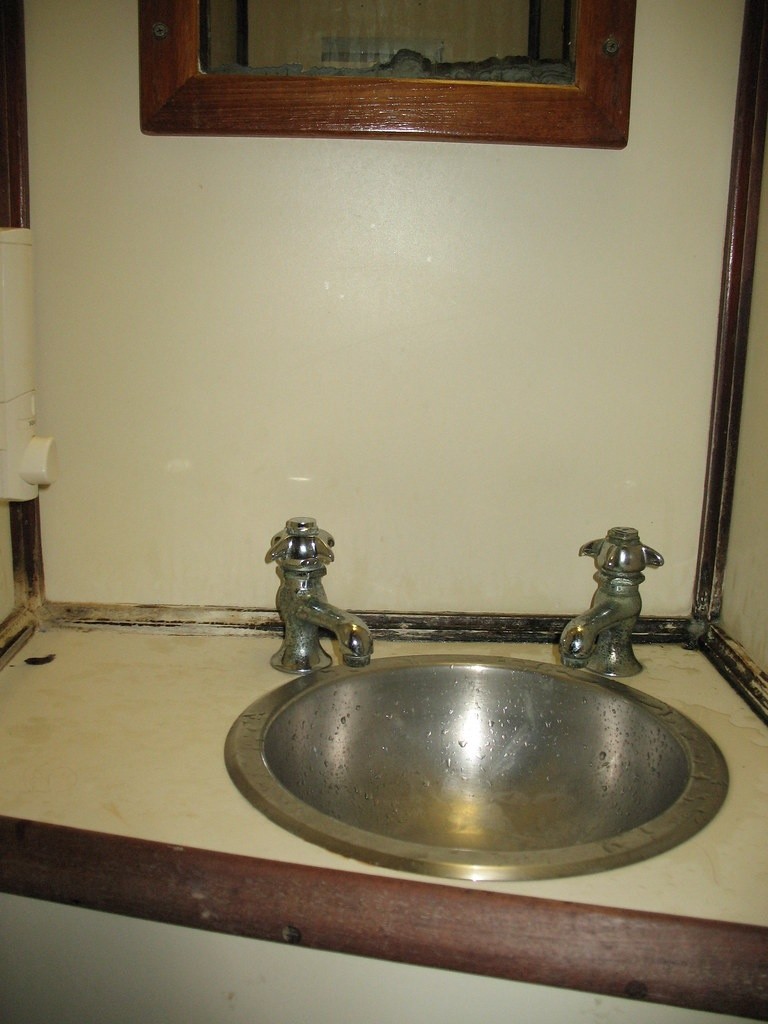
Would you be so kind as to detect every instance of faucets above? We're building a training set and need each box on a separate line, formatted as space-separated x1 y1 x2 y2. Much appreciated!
265 516 374 676
558 527 665 678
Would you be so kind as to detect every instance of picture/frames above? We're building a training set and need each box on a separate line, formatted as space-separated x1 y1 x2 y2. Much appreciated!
138 0 637 150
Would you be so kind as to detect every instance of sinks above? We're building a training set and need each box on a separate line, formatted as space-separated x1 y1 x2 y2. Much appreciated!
223 653 731 883
